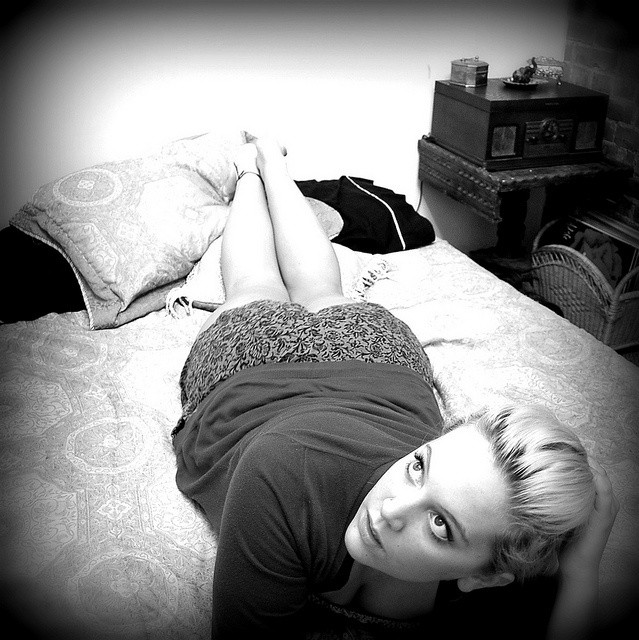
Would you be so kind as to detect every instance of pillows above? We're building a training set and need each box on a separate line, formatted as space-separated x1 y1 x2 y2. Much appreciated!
167 223 392 318
9 128 291 331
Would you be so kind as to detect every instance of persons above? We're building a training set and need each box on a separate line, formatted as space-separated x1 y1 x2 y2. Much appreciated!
170 141 620 639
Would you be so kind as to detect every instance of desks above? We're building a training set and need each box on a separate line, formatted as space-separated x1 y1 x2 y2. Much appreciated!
423 137 606 261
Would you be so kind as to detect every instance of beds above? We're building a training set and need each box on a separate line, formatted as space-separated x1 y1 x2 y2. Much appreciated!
0 178 639 636
